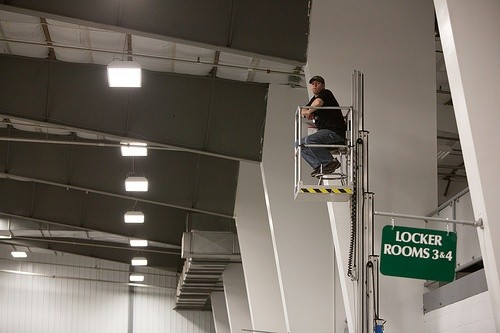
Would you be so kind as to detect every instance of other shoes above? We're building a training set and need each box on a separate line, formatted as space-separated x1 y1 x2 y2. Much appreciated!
311 157 341 179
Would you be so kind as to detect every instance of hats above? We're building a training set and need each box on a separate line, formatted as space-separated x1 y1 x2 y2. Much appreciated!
308 75 324 84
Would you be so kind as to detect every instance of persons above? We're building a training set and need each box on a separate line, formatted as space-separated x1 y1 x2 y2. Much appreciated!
300 76 346 177
